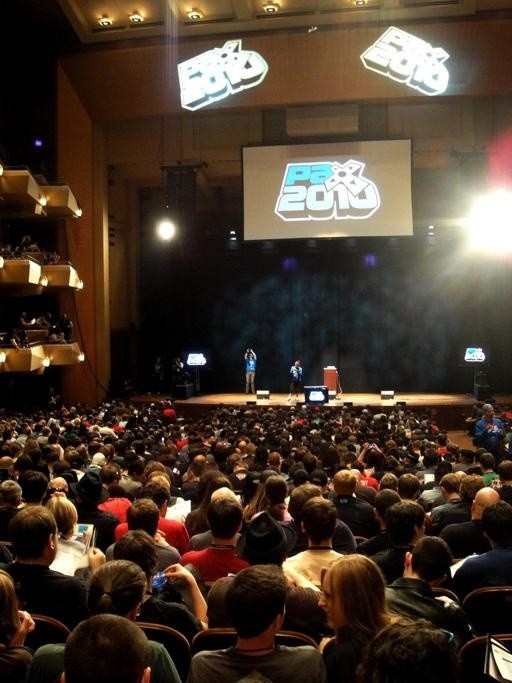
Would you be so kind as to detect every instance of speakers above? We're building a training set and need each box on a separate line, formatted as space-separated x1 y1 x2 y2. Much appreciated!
380 390 394 400
256 390 271 400
175 384 194 399
474 376 496 400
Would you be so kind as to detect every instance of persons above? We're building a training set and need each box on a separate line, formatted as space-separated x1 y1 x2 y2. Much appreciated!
1 398 511 678
124 377 132 391
244 348 257 393
147 356 163 395
288 360 303 398
2 311 73 348
171 357 184 396
0 235 58 263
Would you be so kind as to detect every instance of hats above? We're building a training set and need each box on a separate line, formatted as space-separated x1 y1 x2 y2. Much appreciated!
69 471 110 503
236 511 298 562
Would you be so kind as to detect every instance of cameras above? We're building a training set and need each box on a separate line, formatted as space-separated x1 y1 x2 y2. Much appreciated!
368 444 375 448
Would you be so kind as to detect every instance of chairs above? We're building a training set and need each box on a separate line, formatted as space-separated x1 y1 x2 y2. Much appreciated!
0 539 510 682
